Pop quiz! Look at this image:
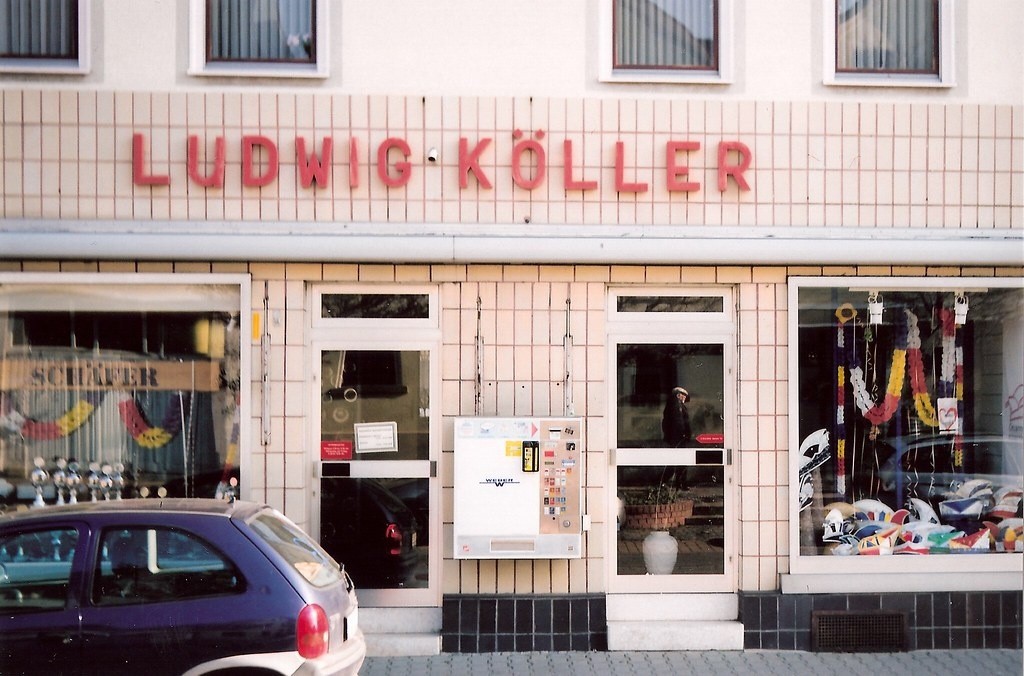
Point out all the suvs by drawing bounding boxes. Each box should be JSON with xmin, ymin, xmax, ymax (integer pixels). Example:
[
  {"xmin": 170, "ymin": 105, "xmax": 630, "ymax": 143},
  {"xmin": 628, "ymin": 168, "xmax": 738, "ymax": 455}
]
[{"xmin": 880, "ymin": 430, "xmax": 1023, "ymax": 505}]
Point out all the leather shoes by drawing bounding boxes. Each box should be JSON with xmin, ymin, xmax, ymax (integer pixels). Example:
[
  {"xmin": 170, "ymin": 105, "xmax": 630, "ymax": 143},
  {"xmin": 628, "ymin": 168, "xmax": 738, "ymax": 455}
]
[
  {"xmin": 661, "ymin": 483, "xmax": 674, "ymax": 491},
  {"xmin": 676, "ymin": 486, "xmax": 690, "ymax": 491}
]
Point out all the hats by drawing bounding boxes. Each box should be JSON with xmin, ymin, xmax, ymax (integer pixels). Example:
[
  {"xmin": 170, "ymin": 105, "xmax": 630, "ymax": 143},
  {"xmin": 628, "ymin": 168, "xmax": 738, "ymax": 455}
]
[{"xmin": 673, "ymin": 387, "xmax": 688, "ymax": 395}]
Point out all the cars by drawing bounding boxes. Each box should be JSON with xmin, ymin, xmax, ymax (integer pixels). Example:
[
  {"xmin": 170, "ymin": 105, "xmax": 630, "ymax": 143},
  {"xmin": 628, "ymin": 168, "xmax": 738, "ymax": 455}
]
[
  {"xmin": 0, "ymin": 499, "xmax": 367, "ymax": 676},
  {"xmin": 102, "ymin": 466, "xmax": 420, "ymax": 590}
]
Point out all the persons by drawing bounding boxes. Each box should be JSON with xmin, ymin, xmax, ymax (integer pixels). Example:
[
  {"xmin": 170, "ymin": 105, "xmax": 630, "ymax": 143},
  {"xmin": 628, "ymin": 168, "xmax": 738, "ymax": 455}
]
[{"xmin": 659, "ymin": 386, "xmax": 693, "ymax": 491}]
